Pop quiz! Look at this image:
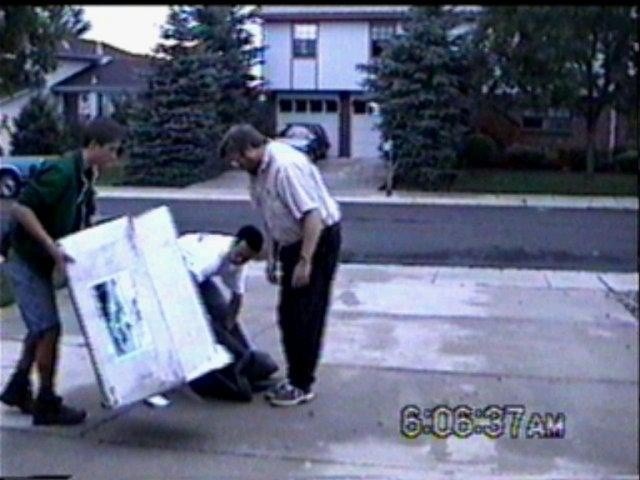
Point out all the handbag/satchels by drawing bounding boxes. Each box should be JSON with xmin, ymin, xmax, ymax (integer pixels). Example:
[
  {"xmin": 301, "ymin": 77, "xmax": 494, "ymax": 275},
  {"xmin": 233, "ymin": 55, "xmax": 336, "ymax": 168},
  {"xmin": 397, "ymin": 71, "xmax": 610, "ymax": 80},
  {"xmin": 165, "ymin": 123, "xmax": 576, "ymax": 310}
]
[{"xmin": 189, "ymin": 324, "xmax": 277, "ymax": 402}]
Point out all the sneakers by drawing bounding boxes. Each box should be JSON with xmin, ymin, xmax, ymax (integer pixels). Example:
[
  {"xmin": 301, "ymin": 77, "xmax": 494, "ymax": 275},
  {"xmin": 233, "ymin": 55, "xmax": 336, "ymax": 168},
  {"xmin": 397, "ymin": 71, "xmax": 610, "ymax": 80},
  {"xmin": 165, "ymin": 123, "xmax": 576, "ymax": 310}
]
[
  {"xmin": 143, "ymin": 395, "xmax": 172, "ymax": 408},
  {"xmin": 264, "ymin": 379, "xmax": 290, "ymax": 399},
  {"xmin": 32, "ymin": 389, "xmax": 86, "ymax": 425},
  {"xmin": 0, "ymin": 373, "xmax": 37, "ymax": 414},
  {"xmin": 271, "ymin": 384, "xmax": 314, "ymax": 407}
]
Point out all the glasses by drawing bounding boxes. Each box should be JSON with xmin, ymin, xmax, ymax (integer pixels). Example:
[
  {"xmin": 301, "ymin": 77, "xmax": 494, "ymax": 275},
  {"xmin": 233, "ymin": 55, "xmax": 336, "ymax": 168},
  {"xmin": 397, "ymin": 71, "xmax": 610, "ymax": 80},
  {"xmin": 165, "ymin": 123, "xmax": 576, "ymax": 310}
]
[{"xmin": 230, "ymin": 153, "xmax": 241, "ymax": 168}]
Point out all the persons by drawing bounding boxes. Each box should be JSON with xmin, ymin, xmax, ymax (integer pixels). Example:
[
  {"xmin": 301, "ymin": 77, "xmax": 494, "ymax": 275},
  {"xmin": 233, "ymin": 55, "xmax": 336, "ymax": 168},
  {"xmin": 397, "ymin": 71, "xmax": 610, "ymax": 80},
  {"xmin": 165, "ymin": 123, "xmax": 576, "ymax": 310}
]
[
  {"xmin": 1, "ymin": 117, "xmax": 123, "ymax": 426},
  {"xmin": 179, "ymin": 225, "xmax": 264, "ymax": 350},
  {"xmin": 218, "ymin": 123, "xmax": 343, "ymax": 408}
]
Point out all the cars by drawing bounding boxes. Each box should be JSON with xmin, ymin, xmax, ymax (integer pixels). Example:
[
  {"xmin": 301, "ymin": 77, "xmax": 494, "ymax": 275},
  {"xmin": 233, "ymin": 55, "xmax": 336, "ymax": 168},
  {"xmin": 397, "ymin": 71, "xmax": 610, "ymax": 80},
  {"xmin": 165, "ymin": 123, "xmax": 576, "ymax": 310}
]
[
  {"xmin": 0, "ymin": 155, "xmax": 62, "ymax": 199},
  {"xmin": 275, "ymin": 122, "xmax": 329, "ymax": 162}
]
